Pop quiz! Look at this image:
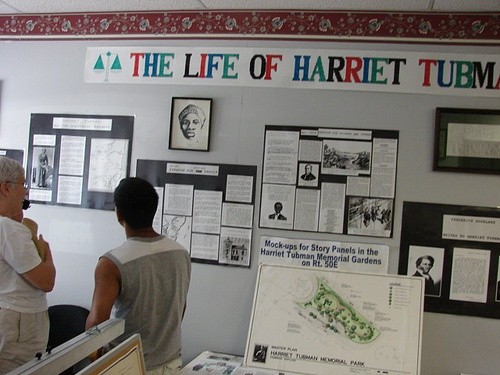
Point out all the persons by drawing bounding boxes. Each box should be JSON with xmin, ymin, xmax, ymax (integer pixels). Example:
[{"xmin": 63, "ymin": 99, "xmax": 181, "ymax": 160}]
[
  {"xmin": 86, "ymin": 177, "xmax": 191, "ymax": 375},
  {"xmin": 413, "ymin": 255, "xmax": 441, "ymax": 296},
  {"xmin": 269, "ymin": 202, "xmax": 287, "ymax": 220},
  {"xmin": 38, "ymin": 148, "xmax": 48, "ymax": 187},
  {"xmin": 349, "ymin": 205, "xmax": 391, "ymax": 227},
  {"xmin": 301, "ymin": 164, "xmax": 316, "ymax": 181},
  {"xmin": 0, "ymin": 156, "xmax": 56, "ymax": 375}
]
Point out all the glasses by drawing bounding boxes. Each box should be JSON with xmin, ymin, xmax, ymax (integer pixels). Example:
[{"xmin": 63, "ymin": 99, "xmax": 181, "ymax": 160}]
[{"xmin": 4, "ymin": 180, "xmax": 28, "ymax": 189}]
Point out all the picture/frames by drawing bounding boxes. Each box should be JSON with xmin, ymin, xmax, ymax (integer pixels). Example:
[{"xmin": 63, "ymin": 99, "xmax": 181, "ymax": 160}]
[{"xmin": 168, "ymin": 97, "xmax": 213, "ymax": 153}]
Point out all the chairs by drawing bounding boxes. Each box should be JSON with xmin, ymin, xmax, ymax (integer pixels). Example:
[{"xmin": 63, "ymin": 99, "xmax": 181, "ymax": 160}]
[{"xmin": 46, "ymin": 305, "xmax": 91, "ymax": 375}]
